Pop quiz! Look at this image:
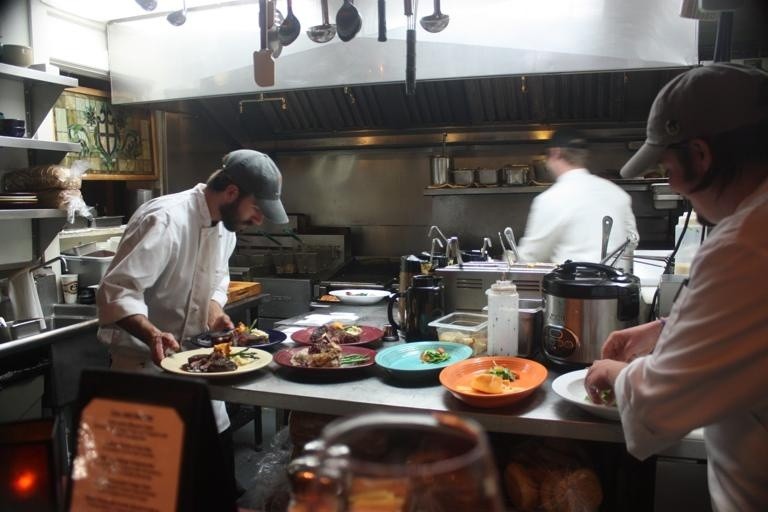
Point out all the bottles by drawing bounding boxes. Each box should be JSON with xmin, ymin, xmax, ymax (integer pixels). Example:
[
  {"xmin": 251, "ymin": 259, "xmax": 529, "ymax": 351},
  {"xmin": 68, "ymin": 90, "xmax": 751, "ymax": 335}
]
[
  {"xmin": 485, "ymin": 281, "xmax": 519, "ymax": 358},
  {"xmin": 673, "ymin": 211, "xmax": 707, "ymax": 275}
]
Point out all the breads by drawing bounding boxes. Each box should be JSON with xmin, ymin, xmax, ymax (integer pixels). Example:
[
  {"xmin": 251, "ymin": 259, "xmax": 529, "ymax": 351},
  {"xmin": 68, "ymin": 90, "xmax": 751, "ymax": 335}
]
[
  {"xmin": 4, "ymin": 165, "xmax": 69, "ymax": 190},
  {"xmin": 35, "ymin": 190, "xmax": 69, "ymax": 208}
]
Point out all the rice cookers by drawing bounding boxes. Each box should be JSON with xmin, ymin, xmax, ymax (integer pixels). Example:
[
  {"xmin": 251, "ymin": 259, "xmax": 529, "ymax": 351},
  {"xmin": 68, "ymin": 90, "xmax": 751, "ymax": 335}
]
[{"xmin": 541, "ymin": 261, "xmax": 641, "ymax": 371}]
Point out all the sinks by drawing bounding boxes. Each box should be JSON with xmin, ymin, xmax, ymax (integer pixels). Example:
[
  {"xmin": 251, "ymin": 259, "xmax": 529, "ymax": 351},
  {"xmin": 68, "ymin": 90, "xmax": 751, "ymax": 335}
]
[{"xmin": 0, "ymin": 317, "xmax": 89, "ymax": 344}]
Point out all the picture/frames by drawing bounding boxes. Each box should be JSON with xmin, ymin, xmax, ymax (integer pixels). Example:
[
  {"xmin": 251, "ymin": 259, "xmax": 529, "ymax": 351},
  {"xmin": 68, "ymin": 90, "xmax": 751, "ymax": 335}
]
[{"xmin": 53, "ymin": 85, "xmax": 159, "ymax": 183}]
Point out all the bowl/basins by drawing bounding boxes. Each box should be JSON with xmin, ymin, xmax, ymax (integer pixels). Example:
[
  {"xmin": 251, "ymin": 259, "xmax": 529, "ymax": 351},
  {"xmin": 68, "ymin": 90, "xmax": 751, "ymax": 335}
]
[
  {"xmin": 1, "ymin": 43, "xmax": 33, "ymax": 68},
  {"xmin": 2, "ymin": 118, "xmax": 25, "ymax": 136}
]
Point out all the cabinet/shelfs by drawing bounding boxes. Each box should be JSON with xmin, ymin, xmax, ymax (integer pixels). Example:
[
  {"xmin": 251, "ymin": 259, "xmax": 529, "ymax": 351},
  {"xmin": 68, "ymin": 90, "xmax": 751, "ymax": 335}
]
[{"xmin": 1, "ymin": 64, "xmax": 94, "ymax": 271}]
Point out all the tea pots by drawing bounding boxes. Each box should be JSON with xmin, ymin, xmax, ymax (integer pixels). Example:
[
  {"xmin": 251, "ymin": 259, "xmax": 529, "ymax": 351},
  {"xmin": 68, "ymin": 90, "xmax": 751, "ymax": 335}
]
[{"xmin": 387, "ymin": 274, "xmax": 444, "ymax": 342}]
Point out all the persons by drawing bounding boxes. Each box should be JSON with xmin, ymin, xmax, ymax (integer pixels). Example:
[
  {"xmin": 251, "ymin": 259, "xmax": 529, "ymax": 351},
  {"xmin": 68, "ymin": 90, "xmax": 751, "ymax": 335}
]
[
  {"xmin": 580, "ymin": 61, "xmax": 767, "ymax": 510},
  {"xmin": 506, "ymin": 125, "xmax": 642, "ymax": 273},
  {"xmin": 95, "ymin": 148, "xmax": 290, "ymax": 512}
]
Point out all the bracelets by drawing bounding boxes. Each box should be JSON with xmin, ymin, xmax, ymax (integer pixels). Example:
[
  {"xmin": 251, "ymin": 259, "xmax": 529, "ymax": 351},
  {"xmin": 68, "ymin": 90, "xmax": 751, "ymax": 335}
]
[{"xmin": 657, "ymin": 317, "xmax": 665, "ymax": 328}]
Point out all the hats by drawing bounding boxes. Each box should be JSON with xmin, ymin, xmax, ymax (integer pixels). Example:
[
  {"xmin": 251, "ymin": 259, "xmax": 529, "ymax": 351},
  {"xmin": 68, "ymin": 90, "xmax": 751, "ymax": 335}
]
[
  {"xmin": 544, "ymin": 128, "xmax": 586, "ymax": 149},
  {"xmin": 222, "ymin": 149, "xmax": 290, "ymax": 225},
  {"xmin": 619, "ymin": 62, "xmax": 767, "ymax": 179}
]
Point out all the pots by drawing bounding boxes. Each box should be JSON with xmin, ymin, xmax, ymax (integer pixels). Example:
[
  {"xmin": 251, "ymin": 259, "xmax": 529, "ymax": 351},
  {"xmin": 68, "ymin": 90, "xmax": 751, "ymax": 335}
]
[{"xmin": 421, "ymin": 150, "xmax": 554, "ymax": 186}]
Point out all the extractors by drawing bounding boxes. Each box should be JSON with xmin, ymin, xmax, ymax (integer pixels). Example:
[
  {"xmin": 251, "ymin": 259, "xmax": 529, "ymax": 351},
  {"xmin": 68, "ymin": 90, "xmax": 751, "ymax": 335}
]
[{"xmin": 105, "ymin": 0, "xmax": 700, "ymax": 152}]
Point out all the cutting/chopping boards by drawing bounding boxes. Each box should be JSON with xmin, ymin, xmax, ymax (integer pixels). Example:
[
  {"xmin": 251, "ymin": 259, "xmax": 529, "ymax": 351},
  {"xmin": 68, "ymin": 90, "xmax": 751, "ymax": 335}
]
[{"xmin": 221, "ymin": 280, "xmax": 262, "ymax": 306}]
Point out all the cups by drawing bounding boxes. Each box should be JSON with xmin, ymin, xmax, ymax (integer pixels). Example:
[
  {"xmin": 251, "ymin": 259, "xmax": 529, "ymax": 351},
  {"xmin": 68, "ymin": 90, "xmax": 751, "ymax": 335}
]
[
  {"xmin": 61, "ymin": 274, "xmax": 80, "ymax": 305},
  {"xmin": 318, "ymin": 412, "xmax": 500, "ymax": 512}
]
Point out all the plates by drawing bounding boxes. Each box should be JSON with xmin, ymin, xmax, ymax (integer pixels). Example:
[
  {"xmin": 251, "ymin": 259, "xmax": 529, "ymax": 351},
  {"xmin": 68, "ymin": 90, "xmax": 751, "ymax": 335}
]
[
  {"xmin": 551, "ymin": 368, "xmax": 621, "ymax": 422},
  {"xmin": 329, "ymin": 289, "xmax": 389, "ymax": 304},
  {"xmin": 273, "ymin": 346, "xmax": 377, "ymax": 373},
  {"xmin": 289, "ymin": 324, "xmax": 384, "ymax": 345},
  {"xmin": 159, "ymin": 346, "xmax": 273, "ymax": 379},
  {"xmin": 437, "ymin": 356, "xmax": 549, "ymax": 406},
  {"xmin": 191, "ymin": 327, "xmax": 286, "ymax": 351},
  {"xmin": 374, "ymin": 341, "xmax": 473, "ymax": 378}
]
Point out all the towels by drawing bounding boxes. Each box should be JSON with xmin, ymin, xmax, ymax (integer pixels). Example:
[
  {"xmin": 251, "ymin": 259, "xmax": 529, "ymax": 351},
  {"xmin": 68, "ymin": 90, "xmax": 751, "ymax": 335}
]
[{"xmin": 5, "ymin": 267, "xmax": 47, "ymax": 330}]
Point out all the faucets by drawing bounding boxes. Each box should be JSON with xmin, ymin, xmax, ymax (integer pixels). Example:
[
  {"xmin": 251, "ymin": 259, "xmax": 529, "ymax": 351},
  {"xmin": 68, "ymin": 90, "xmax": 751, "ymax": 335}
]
[{"xmin": 1, "ymin": 255, "xmax": 68, "ymax": 298}]
